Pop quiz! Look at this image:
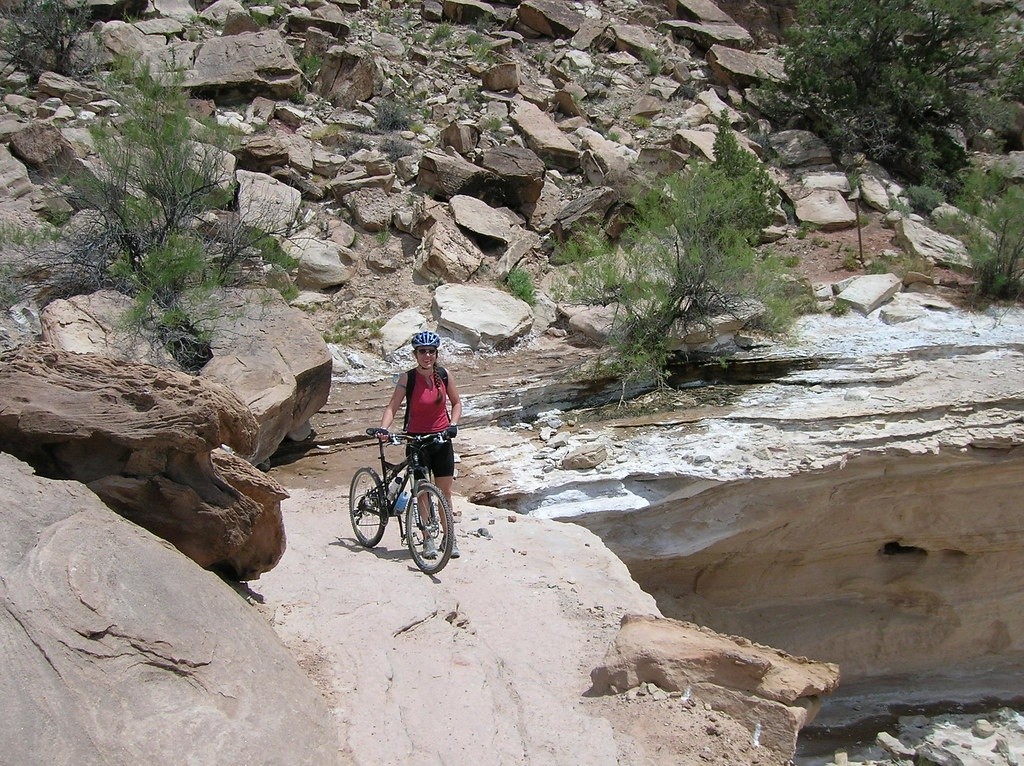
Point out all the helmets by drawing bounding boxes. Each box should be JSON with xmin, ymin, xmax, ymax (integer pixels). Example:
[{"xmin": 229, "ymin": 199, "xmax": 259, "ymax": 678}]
[{"xmin": 412, "ymin": 331, "xmax": 440, "ymax": 350}]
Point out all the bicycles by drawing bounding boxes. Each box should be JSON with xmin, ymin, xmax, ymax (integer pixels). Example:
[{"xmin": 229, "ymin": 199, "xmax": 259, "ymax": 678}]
[{"xmin": 347, "ymin": 428, "xmax": 455, "ymax": 576}]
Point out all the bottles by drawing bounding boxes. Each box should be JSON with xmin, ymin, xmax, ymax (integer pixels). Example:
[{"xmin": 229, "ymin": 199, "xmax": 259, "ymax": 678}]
[
  {"xmin": 394, "ymin": 491, "xmax": 409, "ymax": 514},
  {"xmin": 387, "ymin": 477, "xmax": 402, "ymax": 500}
]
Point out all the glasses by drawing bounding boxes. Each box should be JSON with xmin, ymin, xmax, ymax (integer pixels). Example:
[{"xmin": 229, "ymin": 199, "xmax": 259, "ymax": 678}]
[{"xmin": 414, "ymin": 349, "xmax": 437, "ymax": 354}]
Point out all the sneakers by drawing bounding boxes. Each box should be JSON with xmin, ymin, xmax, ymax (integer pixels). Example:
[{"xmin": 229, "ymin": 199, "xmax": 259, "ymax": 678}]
[
  {"xmin": 422, "ymin": 537, "xmax": 438, "ymax": 560},
  {"xmin": 439, "ymin": 537, "xmax": 460, "ymax": 559}
]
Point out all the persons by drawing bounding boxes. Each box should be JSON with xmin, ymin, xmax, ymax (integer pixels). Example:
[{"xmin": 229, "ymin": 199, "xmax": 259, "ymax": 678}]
[{"xmin": 375, "ymin": 331, "xmax": 462, "ymax": 557}]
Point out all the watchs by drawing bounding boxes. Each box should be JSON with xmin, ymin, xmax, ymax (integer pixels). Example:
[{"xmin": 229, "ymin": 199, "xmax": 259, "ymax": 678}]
[{"xmin": 449, "ymin": 422, "xmax": 457, "ymax": 426}]
